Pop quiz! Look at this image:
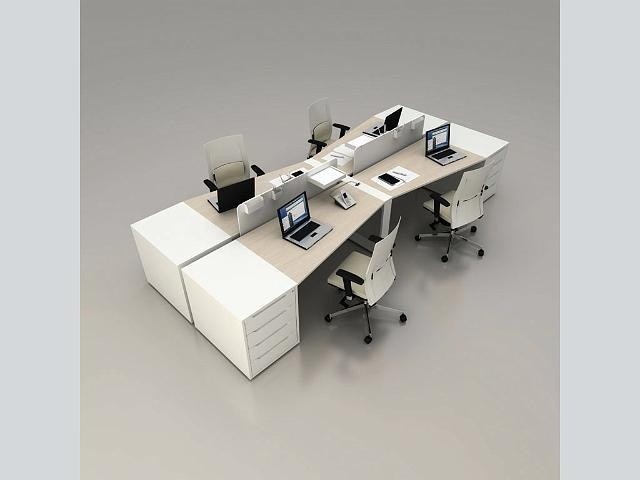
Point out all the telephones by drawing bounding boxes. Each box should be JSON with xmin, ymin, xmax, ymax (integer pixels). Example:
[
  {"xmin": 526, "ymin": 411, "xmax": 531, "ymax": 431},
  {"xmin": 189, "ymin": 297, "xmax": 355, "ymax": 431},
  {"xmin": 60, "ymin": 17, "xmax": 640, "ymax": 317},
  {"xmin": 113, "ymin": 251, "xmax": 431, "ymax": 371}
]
[{"xmin": 330, "ymin": 188, "xmax": 356, "ymax": 210}]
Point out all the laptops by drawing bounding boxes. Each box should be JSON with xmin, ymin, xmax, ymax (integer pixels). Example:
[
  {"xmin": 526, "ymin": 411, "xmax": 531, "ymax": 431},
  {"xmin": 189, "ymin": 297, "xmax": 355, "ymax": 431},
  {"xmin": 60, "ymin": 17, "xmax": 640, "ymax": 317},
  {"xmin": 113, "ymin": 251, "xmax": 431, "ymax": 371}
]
[
  {"xmin": 276, "ymin": 190, "xmax": 334, "ymax": 250},
  {"xmin": 425, "ymin": 122, "xmax": 468, "ymax": 166},
  {"xmin": 207, "ymin": 176, "xmax": 256, "ymax": 214},
  {"xmin": 362, "ymin": 106, "xmax": 403, "ymax": 137}
]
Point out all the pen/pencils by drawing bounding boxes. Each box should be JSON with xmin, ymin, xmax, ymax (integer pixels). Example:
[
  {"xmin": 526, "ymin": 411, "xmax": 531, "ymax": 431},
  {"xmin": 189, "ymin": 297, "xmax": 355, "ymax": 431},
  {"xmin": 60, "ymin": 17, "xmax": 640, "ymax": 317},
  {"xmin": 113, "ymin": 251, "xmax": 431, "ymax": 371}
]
[
  {"xmin": 392, "ymin": 171, "xmax": 406, "ymax": 176},
  {"xmin": 331, "ymin": 155, "xmax": 344, "ymax": 158}
]
